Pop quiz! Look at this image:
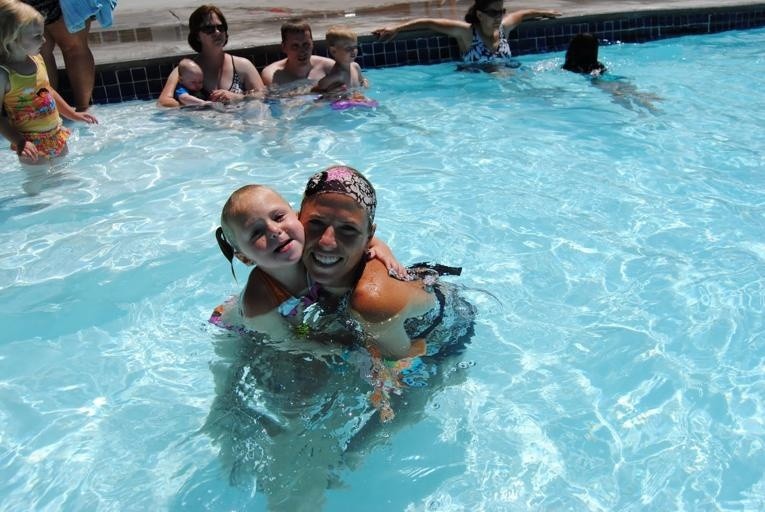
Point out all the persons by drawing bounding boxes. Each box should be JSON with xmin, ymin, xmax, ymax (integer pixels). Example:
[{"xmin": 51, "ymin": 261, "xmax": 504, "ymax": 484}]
[
  {"xmin": 159, "ymin": 5, "xmax": 266, "ymax": 107},
  {"xmin": 376, "ymin": 2, "xmax": 563, "ymax": 74},
  {"xmin": 559, "ymin": 36, "xmax": 668, "ymax": 123},
  {"xmin": 172, "ymin": 58, "xmax": 216, "ymax": 109},
  {"xmin": 38, "ymin": 1, "xmax": 117, "ymax": 110},
  {"xmin": 295, "ymin": 163, "xmax": 476, "ymax": 357},
  {"xmin": 315, "ymin": 27, "xmax": 370, "ymax": 93},
  {"xmin": 260, "ymin": 18, "xmax": 349, "ymax": 97},
  {"xmin": 1, "ymin": 1, "xmax": 99, "ymax": 166},
  {"xmin": 208, "ymin": 187, "xmax": 398, "ymax": 424}
]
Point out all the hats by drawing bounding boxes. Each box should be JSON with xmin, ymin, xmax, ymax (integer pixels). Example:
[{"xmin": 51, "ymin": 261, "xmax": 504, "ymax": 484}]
[{"xmin": 303, "ymin": 165, "xmax": 378, "ymax": 228}]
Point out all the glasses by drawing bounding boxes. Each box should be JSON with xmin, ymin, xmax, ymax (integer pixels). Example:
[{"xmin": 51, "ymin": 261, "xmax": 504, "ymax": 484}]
[
  {"xmin": 199, "ymin": 23, "xmax": 229, "ymax": 34},
  {"xmin": 478, "ymin": 6, "xmax": 506, "ymax": 18}
]
